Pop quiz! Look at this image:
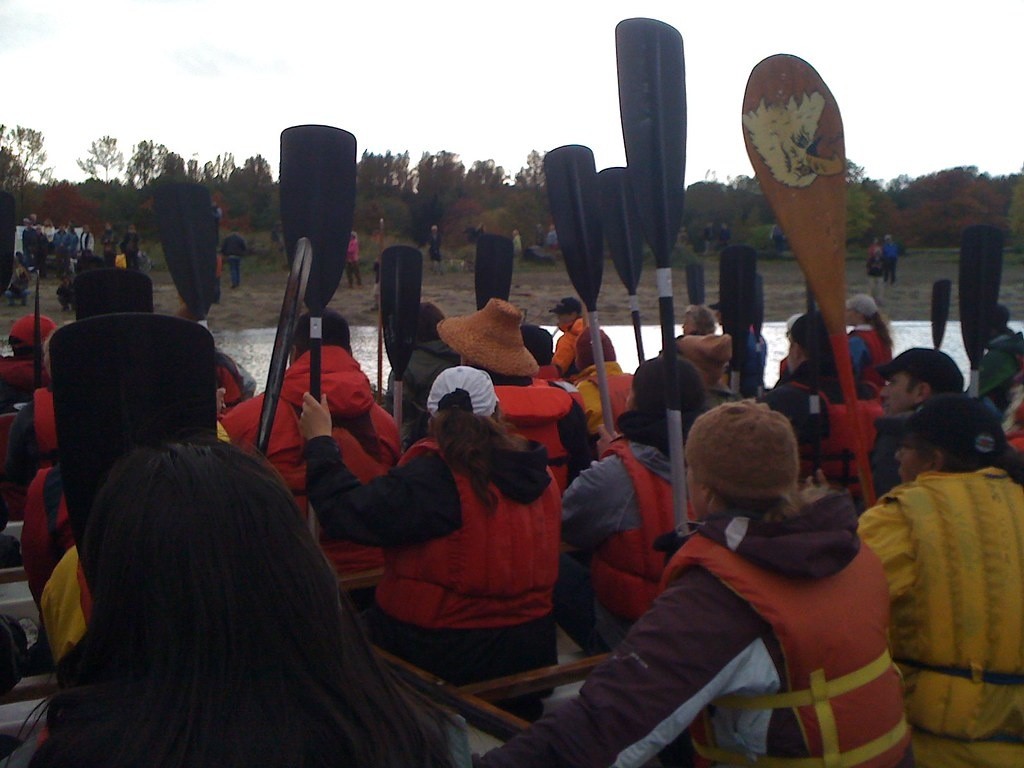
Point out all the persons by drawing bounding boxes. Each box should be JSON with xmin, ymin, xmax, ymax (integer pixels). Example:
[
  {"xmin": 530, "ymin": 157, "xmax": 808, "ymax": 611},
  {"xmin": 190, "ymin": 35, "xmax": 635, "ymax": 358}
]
[
  {"xmin": 427, "ymin": 225, "xmax": 444, "ymax": 272},
  {"xmin": 5, "ymin": 210, "xmax": 140, "ymax": 312},
  {"xmin": 346, "ymin": 231, "xmax": 363, "ymax": 289},
  {"xmin": 535, "ymin": 223, "xmax": 558, "ymax": 250},
  {"xmin": 510, "ymin": 227, "xmax": 524, "ymax": 267},
  {"xmin": 461, "ymin": 220, "xmax": 488, "ymax": 244},
  {"xmin": 865, "ymin": 234, "xmax": 899, "ymax": 285},
  {"xmin": 0, "ymin": 293, "xmax": 1024, "ymax": 768},
  {"xmin": 214, "ymin": 226, "xmax": 246, "ymax": 305},
  {"xmin": 771, "ymin": 218, "xmax": 785, "ymax": 251},
  {"xmin": 702, "ymin": 221, "xmax": 731, "ymax": 256}
]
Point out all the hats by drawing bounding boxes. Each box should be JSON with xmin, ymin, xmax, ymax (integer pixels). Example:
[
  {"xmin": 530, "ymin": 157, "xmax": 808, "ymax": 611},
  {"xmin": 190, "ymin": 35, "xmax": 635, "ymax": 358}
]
[
  {"xmin": 549, "ymin": 297, "xmax": 582, "ymax": 313},
  {"xmin": 792, "ymin": 310, "xmax": 827, "ymax": 345},
  {"xmin": 576, "ymin": 326, "xmax": 616, "ymax": 370},
  {"xmin": 876, "ymin": 348, "xmax": 964, "ymax": 393},
  {"xmin": 993, "ymin": 303, "xmax": 1008, "ymax": 323},
  {"xmin": 416, "ymin": 303, "xmax": 445, "ymax": 340},
  {"xmin": 521, "ymin": 325, "xmax": 553, "ymax": 364},
  {"xmin": 632, "ymin": 354, "xmax": 702, "ymax": 414},
  {"xmin": 885, "ymin": 235, "xmax": 891, "ymax": 240},
  {"xmin": 677, "ymin": 333, "xmax": 731, "ymax": 386},
  {"xmin": 9, "ymin": 315, "xmax": 58, "ymax": 348},
  {"xmin": 437, "ymin": 298, "xmax": 540, "ymax": 375},
  {"xmin": 294, "ymin": 307, "xmax": 351, "ymax": 343},
  {"xmin": 845, "ymin": 294, "xmax": 877, "ymax": 318},
  {"xmin": 874, "ymin": 394, "xmax": 1006, "ymax": 454},
  {"xmin": 428, "ymin": 366, "xmax": 499, "ymax": 416},
  {"xmin": 686, "ymin": 397, "xmax": 800, "ymax": 499}
]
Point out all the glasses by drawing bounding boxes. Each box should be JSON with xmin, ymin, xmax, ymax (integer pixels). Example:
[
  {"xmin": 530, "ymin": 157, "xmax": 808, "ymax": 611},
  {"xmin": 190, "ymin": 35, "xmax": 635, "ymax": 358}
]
[{"xmin": 786, "ymin": 330, "xmax": 791, "ymax": 337}]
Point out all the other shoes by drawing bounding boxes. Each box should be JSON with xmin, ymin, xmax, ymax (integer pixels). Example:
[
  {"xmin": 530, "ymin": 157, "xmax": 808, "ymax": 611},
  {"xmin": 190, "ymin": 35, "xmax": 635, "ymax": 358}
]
[{"xmin": 14, "ymin": 642, "xmax": 55, "ymax": 677}]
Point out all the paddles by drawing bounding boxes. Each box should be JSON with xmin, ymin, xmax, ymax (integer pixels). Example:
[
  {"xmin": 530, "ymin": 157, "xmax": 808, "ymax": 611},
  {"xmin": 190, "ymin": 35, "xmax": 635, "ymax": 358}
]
[
  {"xmin": 931, "ymin": 280, "xmax": 951, "ymax": 351},
  {"xmin": 153, "ymin": 181, "xmax": 218, "ymax": 328},
  {"xmin": 255, "ymin": 237, "xmax": 314, "ymax": 458},
  {"xmin": 474, "ymin": 234, "xmax": 514, "ymax": 310},
  {"xmin": 0, "ymin": 191, "xmax": 16, "ymax": 295},
  {"xmin": 741, "ymin": 53, "xmax": 877, "ymax": 507},
  {"xmin": 279, "ymin": 124, "xmax": 357, "ymax": 544},
  {"xmin": 751, "ymin": 274, "xmax": 764, "ymax": 398},
  {"xmin": 73, "ymin": 267, "xmax": 153, "ymax": 321},
  {"xmin": 543, "ymin": 145, "xmax": 615, "ymax": 439},
  {"xmin": 380, "ymin": 245, "xmax": 423, "ymax": 434},
  {"xmin": 806, "ymin": 280, "xmax": 821, "ymax": 488},
  {"xmin": 49, "ymin": 313, "xmax": 218, "ymax": 604},
  {"xmin": 719, "ymin": 245, "xmax": 757, "ymax": 394},
  {"xmin": 958, "ymin": 223, "xmax": 1004, "ymax": 398},
  {"xmin": 597, "ymin": 167, "xmax": 646, "ymax": 366},
  {"xmin": 685, "ymin": 262, "xmax": 705, "ymax": 305},
  {"xmin": 34, "ymin": 276, "xmax": 41, "ymax": 388},
  {"xmin": 614, "ymin": 16, "xmax": 687, "ymax": 538}
]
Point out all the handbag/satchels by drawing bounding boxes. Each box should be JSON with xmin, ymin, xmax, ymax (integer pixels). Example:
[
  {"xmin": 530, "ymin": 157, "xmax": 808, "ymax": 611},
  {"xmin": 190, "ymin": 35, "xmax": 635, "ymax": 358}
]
[{"xmin": 114, "ymin": 253, "xmax": 127, "ymax": 269}]
